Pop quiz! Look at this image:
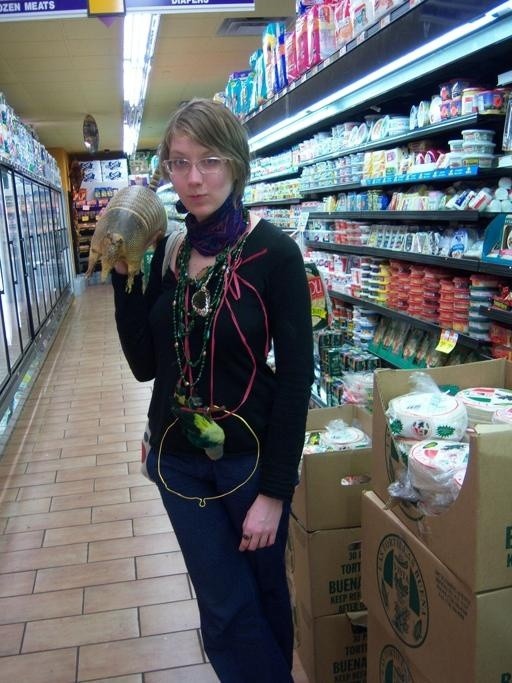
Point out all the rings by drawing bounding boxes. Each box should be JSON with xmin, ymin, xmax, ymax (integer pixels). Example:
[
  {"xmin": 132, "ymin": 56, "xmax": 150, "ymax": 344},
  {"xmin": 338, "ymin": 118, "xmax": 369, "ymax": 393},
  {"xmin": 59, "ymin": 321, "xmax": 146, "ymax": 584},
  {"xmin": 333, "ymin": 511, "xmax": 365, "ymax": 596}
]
[{"xmin": 241, "ymin": 533, "xmax": 250, "ymax": 541}]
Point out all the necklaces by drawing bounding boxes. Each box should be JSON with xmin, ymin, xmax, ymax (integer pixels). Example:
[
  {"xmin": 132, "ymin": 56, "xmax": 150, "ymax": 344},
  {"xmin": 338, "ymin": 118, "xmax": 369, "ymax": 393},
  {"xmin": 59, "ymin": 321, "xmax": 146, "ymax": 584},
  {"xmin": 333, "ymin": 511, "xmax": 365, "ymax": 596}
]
[{"xmin": 172, "ymin": 206, "xmax": 252, "ymax": 393}]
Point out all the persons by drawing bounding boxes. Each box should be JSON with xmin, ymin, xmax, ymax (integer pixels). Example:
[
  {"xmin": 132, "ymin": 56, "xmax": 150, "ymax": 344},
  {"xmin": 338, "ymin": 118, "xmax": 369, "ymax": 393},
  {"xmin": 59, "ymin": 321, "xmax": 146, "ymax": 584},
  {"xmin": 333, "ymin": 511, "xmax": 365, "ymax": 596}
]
[{"xmin": 109, "ymin": 94, "xmax": 320, "ymax": 682}]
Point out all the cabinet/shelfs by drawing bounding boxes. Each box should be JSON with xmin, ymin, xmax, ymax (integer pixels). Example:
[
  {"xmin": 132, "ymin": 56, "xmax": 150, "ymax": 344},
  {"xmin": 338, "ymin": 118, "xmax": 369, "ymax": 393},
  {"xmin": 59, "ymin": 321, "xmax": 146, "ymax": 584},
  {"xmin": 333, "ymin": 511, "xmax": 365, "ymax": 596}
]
[
  {"xmin": 0, "ymin": 157, "xmax": 80, "ymax": 459},
  {"xmin": 72, "ymin": 185, "xmax": 119, "ymax": 283}
]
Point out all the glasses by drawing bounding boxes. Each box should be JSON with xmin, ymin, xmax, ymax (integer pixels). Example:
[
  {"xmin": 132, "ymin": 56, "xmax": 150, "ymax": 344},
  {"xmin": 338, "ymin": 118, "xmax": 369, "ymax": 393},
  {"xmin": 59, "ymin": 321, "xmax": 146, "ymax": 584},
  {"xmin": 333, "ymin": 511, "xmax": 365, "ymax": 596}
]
[{"xmin": 162, "ymin": 156, "xmax": 228, "ymax": 175}]
